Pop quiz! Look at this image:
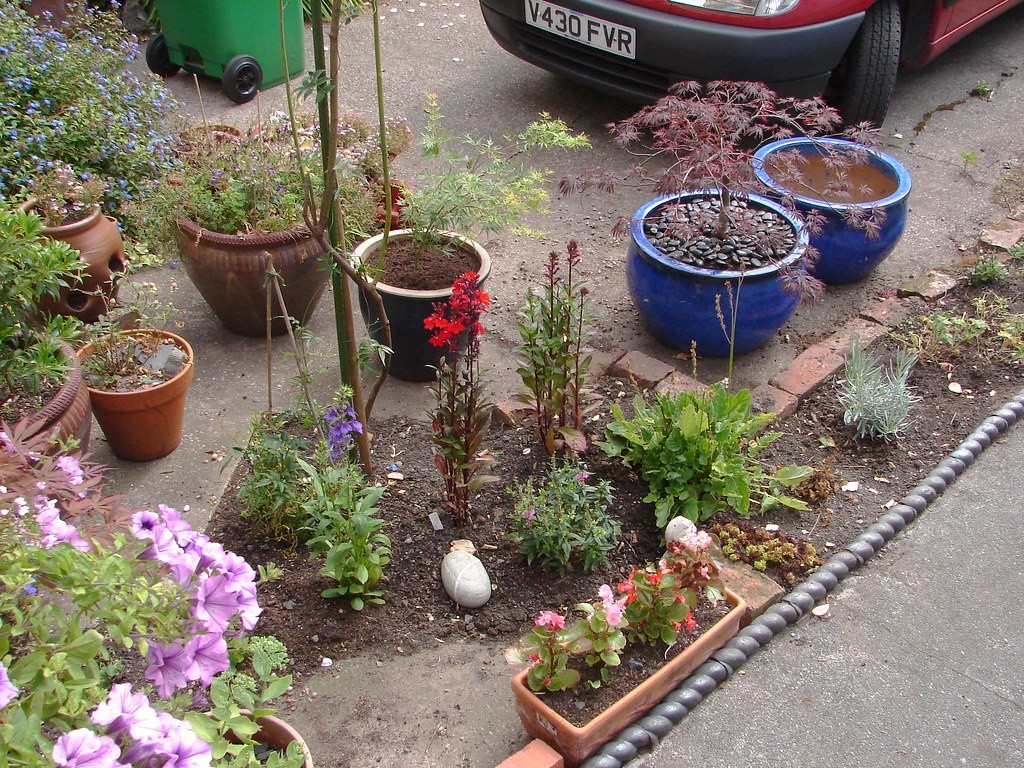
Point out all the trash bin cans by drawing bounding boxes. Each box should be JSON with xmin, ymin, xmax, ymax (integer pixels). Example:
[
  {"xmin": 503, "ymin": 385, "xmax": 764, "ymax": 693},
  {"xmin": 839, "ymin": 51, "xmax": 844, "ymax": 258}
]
[{"xmin": 145, "ymin": 0, "xmax": 305, "ymax": 102}]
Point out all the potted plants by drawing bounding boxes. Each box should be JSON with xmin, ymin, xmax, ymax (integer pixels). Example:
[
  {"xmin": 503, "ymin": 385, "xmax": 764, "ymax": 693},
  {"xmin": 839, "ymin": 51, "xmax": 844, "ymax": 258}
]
[
  {"xmin": 0, "ymin": 208, "xmax": 95, "ymax": 475},
  {"xmin": 358, "ymin": 90, "xmax": 594, "ymax": 380},
  {"xmin": 559, "ymin": 79, "xmax": 884, "ymax": 360}
]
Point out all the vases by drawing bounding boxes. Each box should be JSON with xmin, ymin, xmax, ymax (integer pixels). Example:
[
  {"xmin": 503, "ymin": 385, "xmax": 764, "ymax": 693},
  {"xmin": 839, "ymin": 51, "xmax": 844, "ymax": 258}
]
[
  {"xmin": 510, "ymin": 571, "xmax": 748, "ymax": 764},
  {"xmin": 192, "ymin": 708, "xmax": 315, "ymax": 768},
  {"xmin": 18, "ymin": 191, "xmax": 127, "ymax": 326},
  {"xmin": 170, "ymin": 198, "xmax": 334, "ymax": 338},
  {"xmin": 752, "ymin": 137, "xmax": 911, "ymax": 287},
  {"xmin": 77, "ymin": 328, "xmax": 194, "ymax": 462}
]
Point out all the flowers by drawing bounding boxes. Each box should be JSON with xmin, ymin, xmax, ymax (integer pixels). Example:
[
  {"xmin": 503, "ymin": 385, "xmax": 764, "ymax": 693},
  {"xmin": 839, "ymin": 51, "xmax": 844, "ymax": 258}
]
[
  {"xmin": 522, "ymin": 522, "xmax": 718, "ymax": 691},
  {"xmin": 20, "ymin": 162, "xmax": 112, "ymax": 226}
]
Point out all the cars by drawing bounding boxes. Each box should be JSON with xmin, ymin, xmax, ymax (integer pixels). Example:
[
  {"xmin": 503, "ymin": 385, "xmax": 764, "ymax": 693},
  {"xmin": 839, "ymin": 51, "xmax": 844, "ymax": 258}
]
[{"xmin": 479, "ymin": 0, "xmax": 1023, "ymax": 144}]
